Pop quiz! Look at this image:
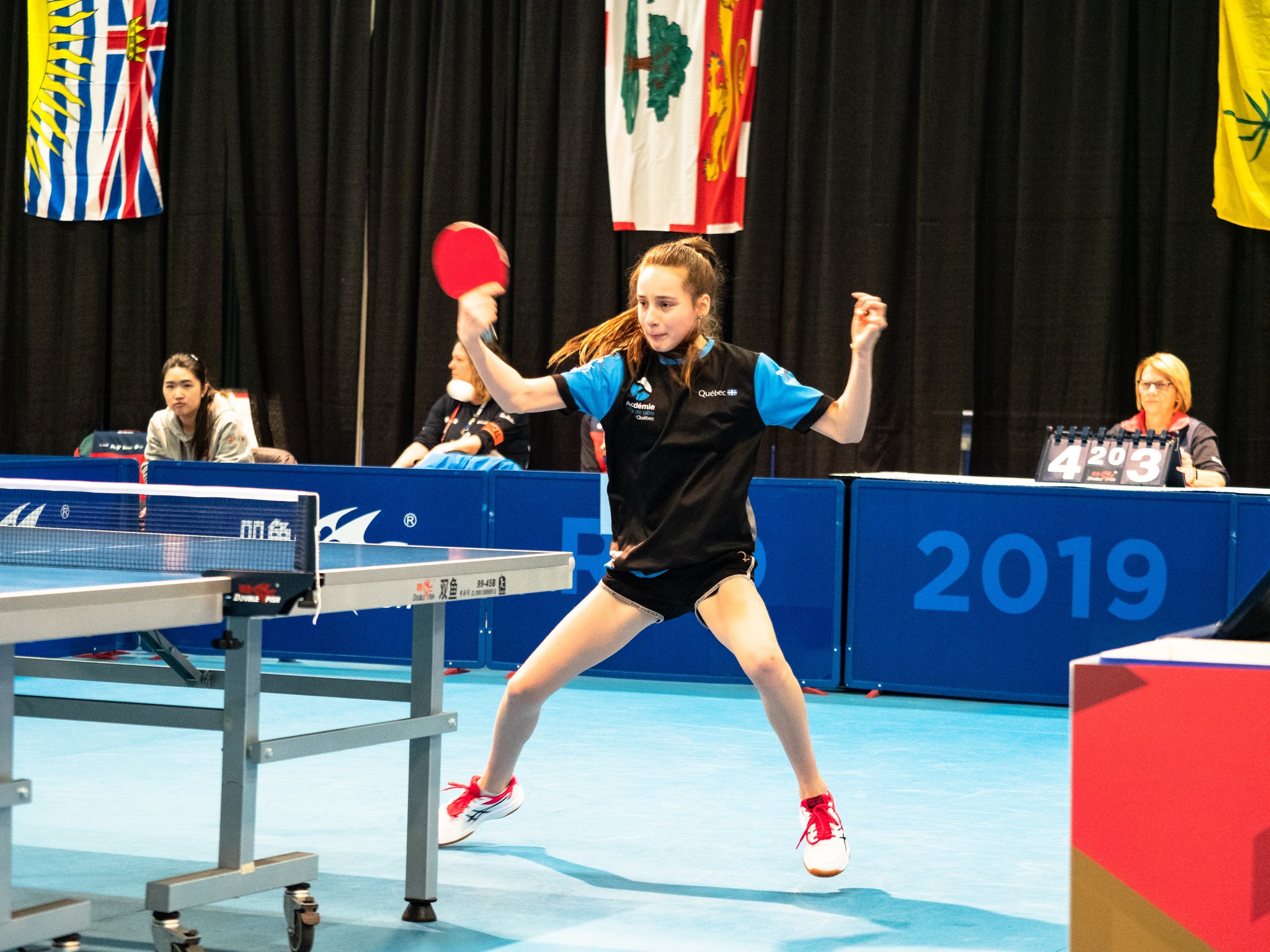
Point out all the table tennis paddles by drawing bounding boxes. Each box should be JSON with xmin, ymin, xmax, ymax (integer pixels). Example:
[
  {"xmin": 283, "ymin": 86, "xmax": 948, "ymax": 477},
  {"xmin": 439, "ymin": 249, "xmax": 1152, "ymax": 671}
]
[{"xmin": 430, "ymin": 219, "xmax": 512, "ymax": 345}]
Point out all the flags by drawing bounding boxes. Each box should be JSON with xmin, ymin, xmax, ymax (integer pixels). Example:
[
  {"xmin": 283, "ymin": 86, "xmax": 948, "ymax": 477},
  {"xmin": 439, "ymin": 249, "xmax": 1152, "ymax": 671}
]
[
  {"xmin": 604, "ymin": 0, "xmax": 763, "ymax": 235},
  {"xmin": 1210, "ymin": 0, "xmax": 1270, "ymax": 230},
  {"xmin": 23, "ymin": 0, "xmax": 167, "ymax": 221}
]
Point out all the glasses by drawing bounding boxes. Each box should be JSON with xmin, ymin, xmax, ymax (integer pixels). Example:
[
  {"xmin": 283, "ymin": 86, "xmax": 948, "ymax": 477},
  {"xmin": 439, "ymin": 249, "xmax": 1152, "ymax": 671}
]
[{"xmin": 1137, "ymin": 380, "xmax": 1174, "ymax": 391}]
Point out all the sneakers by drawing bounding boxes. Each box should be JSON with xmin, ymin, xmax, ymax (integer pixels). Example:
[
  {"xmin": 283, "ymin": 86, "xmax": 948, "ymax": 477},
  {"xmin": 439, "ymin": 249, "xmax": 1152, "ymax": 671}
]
[
  {"xmin": 438, "ymin": 774, "xmax": 524, "ymax": 846},
  {"xmin": 800, "ymin": 790, "xmax": 851, "ymax": 878}
]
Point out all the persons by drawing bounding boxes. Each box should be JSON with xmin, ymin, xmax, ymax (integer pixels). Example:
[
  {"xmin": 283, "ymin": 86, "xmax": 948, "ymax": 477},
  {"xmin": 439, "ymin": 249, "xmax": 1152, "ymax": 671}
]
[
  {"xmin": 437, "ymin": 235, "xmax": 887, "ymax": 877},
  {"xmin": 139, "ymin": 352, "xmax": 254, "ymax": 532},
  {"xmin": 390, "ymin": 338, "xmax": 528, "ymax": 470},
  {"xmin": 579, "ymin": 415, "xmax": 608, "ymax": 473},
  {"xmin": 1103, "ymin": 352, "xmax": 1230, "ymax": 488}
]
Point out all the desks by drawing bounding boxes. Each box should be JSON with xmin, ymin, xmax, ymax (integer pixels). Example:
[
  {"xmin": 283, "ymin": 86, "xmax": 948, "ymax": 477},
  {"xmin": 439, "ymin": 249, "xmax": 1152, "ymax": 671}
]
[{"xmin": 0, "ymin": 446, "xmax": 1269, "ymax": 706}]
